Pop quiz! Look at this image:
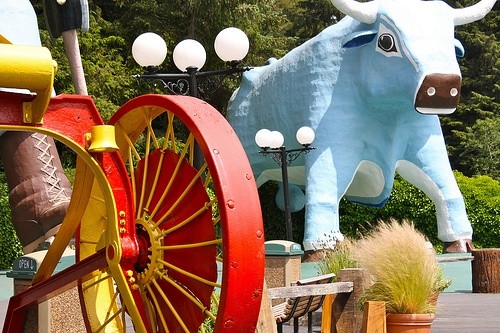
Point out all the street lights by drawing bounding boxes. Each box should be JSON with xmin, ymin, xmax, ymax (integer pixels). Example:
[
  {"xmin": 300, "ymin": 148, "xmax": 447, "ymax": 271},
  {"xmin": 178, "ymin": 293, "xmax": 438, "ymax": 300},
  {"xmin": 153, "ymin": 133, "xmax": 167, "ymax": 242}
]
[
  {"xmin": 255, "ymin": 125, "xmax": 317, "ymax": 243},
  {"xmin": 130, "ymin": 27, "xmax": 255, "ymax": 185}
]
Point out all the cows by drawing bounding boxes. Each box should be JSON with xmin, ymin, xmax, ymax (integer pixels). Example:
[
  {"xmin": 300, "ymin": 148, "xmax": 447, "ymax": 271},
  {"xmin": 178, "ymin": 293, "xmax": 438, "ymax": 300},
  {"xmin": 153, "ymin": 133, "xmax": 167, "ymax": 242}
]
[{"xmin": 217, "ymin": 0, "xmax": 497, "ymax": 264}]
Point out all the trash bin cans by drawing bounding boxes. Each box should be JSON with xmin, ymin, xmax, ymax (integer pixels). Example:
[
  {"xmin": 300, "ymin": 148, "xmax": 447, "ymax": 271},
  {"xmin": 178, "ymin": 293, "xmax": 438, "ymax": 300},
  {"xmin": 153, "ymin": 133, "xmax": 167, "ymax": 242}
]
[
  {"xmin": 263, "ymin": 239, "xmax": 305, "ymax": 307},
  {"xmin": 4, "ymin": 248, "xmax": 77, "ymax": 332}
]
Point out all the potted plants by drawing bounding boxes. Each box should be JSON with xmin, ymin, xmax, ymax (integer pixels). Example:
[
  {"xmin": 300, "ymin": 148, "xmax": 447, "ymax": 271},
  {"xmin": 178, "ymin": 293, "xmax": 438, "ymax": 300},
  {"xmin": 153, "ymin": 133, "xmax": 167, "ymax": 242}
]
[{"xmin": 308, "ymin": 217, "xmax": 451, "ymax": 333}]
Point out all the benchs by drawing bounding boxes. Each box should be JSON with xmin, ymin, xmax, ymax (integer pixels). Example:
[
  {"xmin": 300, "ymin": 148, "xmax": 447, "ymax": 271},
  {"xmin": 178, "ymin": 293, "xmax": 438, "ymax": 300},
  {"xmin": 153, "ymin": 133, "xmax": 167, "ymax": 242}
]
[{"xmin": 272, "ymin": 273, "xmax": 336, "ymax": 333}]
